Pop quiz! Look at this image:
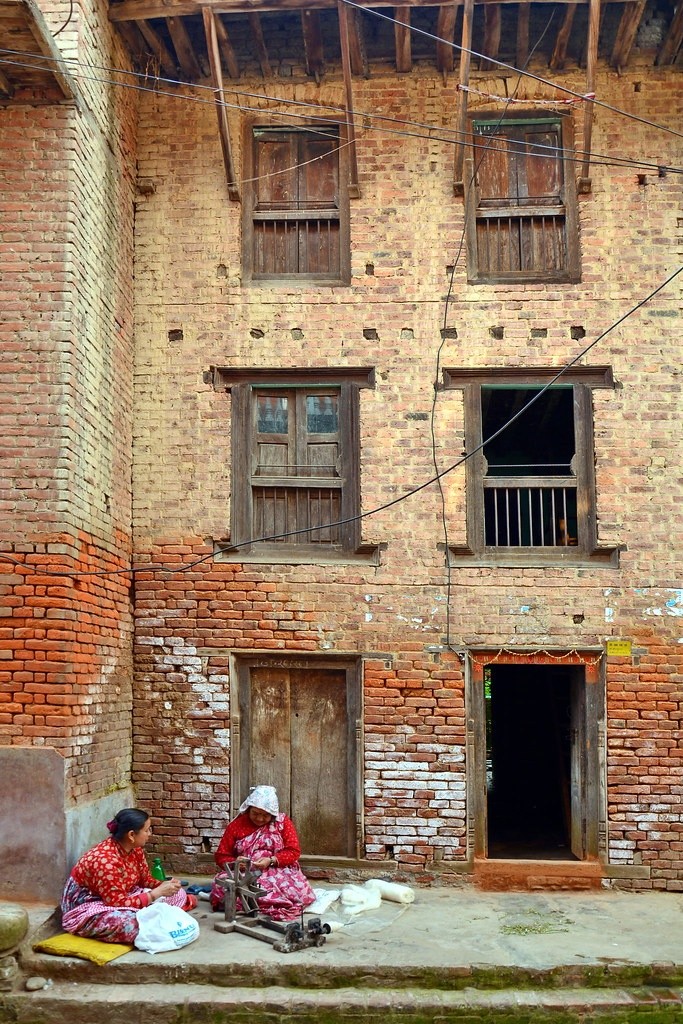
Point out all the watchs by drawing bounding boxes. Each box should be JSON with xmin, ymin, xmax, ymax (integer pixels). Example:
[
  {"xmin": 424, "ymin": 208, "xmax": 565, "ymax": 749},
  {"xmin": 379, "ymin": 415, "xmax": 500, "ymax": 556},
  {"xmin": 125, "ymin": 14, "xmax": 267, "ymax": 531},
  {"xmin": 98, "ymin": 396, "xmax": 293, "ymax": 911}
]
[{"xmin": 269, "ymin": 856, "xmax": 274, "ymax": 866}]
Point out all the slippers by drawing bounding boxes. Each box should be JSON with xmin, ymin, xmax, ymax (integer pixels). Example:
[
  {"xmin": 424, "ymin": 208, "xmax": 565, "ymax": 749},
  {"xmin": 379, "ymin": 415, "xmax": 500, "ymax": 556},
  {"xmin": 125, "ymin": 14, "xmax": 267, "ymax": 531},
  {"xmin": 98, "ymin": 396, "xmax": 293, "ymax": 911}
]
[
  {"xmin": 166, "ymin": 877, "xmax": 189, "ymax": 886},
  {"xmin": 186, "ymin": 885, "xmax": 212, "ymax": 894}
]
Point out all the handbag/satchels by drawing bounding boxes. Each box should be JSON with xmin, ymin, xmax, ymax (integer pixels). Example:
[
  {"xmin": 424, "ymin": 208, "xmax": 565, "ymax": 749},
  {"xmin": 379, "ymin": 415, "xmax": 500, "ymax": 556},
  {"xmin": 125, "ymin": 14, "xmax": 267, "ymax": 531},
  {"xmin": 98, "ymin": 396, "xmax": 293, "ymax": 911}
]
[{"xmin": 134, "ymin": 902, "xmax": 200, "ymax": 956}]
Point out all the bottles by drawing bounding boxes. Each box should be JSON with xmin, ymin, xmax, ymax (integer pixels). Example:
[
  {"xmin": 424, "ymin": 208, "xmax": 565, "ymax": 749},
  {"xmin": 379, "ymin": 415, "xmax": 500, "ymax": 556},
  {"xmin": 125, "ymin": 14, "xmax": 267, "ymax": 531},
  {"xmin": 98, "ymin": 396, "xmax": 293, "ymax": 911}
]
[{"xmin": 152, "ymin": 858, "xmax": 165, "ymax": 881}]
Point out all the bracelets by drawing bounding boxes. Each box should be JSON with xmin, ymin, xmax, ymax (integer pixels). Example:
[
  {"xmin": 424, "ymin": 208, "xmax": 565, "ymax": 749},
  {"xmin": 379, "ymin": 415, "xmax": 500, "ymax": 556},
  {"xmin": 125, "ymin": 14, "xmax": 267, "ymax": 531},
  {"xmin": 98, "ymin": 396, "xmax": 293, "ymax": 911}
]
[{"xmin": 148, "ymin": 890, "xmax": 156, "ymax": 902}]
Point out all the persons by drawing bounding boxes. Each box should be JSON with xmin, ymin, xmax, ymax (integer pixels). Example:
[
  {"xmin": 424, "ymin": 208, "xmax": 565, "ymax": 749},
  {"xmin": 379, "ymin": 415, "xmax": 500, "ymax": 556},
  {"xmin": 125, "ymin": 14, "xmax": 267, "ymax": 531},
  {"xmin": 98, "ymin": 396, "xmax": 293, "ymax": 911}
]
[
  {"xmin": 62, "ymin": 807, "xmax": 188, "ymax": 946},
  {"xmin": 210, "ymin": 786, "xmax": 316, "ymax": 921}
]
